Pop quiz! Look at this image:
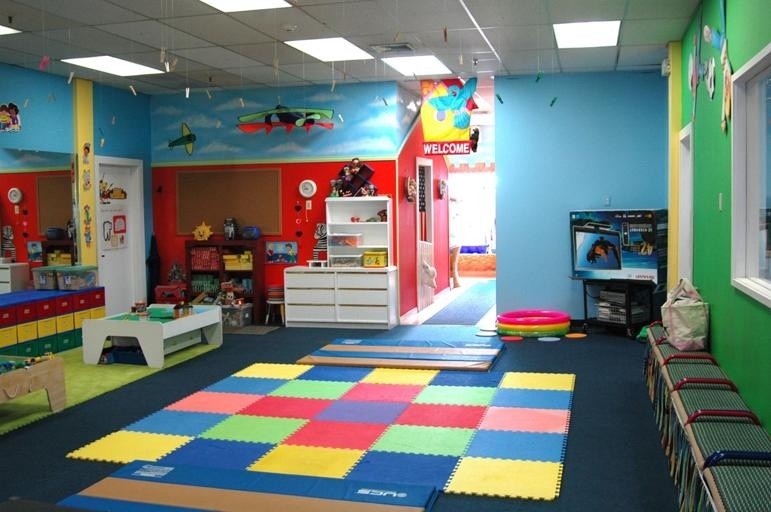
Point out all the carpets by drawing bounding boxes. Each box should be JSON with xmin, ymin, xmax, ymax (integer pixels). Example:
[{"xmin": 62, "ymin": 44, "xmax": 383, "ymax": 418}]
[{"xmin": 0, "ymin": 340, "xmax": 225, "ymax": 436}]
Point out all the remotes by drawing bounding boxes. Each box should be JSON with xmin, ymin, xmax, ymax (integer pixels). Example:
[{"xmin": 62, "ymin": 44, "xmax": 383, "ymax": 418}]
[{"xmin": 622, "ymin": 222, "xmax": 629, "ymax": 246}]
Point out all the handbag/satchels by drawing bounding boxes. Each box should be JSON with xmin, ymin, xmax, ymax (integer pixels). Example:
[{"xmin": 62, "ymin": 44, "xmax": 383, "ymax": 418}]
[{"xmin": 661, "ymin": 278, "xmax": 710, "ymax": 351}]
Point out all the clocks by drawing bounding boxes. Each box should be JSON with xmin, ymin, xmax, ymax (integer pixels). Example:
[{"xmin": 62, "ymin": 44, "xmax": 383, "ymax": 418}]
[
  {"xmin": 8, "ymin": 188, "xmax": 23, "ymax": 204},
  {"xmin": 299, "ymin": 178, "xmax": 318, "ymax": 198}
]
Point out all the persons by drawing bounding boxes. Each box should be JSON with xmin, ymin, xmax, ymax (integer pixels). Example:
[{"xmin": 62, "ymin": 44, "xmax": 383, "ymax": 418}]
[{"xmin": 329, "ymin": 157, "xmax": 377, "ymax": 197}]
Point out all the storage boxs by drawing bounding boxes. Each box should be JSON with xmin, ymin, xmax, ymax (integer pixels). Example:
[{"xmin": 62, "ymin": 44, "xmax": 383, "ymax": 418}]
[
  {"xmin": 219, "ymin": 303, "xmax": 254, "ymax": 326},
  {"xmin": 31, "ymin": 265, "xmax": 58, "ymax": 291},
  {"xmin": 569, "ymin": 210, "xmax": 669, "ymax": 284},
  {"xmin": 55, "ymin": 265, "xmax": 99, "ymax": 292}
]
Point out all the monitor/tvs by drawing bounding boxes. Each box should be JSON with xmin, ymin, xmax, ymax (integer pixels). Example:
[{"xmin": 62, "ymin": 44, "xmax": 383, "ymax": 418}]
[{"xmin": 572, "ymin": 223, "xmax": 621, "ymax": 270}]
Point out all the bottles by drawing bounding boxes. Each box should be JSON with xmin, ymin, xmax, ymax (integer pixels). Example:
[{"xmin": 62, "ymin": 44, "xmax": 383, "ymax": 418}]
[
  {"xmin": 382, "ymin": 215, "xmax": 387, "ymax": 221},
  {"xmin": 224, "ymin": 218, "xmax": 237, "ymax": 240},
  {"xmin": 239, "ymin": 226, "xmax": 261, "ymax": 241}
]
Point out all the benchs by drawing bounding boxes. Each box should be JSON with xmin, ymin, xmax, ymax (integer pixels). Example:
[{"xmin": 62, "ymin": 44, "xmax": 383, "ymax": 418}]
[{"xmin": 644, "ymin": 321, "xmax": 771, "ymax": 511}]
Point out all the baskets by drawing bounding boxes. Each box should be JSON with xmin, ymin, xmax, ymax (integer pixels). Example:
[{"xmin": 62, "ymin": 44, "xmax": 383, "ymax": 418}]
[{"xmin": 594, "ymin": 302, "xmax": 651, "ymax": 325}]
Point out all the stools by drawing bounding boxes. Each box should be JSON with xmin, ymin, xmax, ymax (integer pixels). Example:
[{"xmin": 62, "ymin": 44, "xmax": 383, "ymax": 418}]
[{"xmin": 266, "ymin": 286, "xmax": 286, "ymax": 328}]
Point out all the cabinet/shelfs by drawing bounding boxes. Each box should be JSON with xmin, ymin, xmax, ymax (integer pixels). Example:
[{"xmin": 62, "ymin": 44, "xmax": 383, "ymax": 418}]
[
  {"xmin": 185, "ymin": 239, "xmax": 266, "ymax": 323},
  {"xmin": 0, "ymin": 288, "xmax": 106, "ymax": 374},
  {"xmin": 282, "ymin": 196, "xmax": 397, "ymax": 329},
  {"xmin": 0, "ymin": 262, "xmax": 29, "ymax": 293}
]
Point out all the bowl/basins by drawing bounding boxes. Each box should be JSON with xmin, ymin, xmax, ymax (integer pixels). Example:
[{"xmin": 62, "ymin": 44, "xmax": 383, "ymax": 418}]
[{"xmin": 637, "ymin": 325, "xmax": 650, "ymax": 341}]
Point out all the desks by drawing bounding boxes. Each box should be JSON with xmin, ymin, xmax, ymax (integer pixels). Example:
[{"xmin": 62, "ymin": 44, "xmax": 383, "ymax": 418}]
[{"xmin": 0, "ymin": 303, "xmax": 224, "ymax": 411}]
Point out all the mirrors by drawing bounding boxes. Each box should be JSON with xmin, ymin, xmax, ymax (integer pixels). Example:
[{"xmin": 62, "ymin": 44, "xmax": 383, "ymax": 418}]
[{"xmin": 1, "ymin": 148, "xmax": 78, "ymax": 264}]
